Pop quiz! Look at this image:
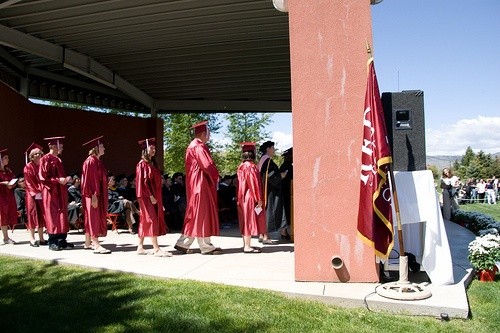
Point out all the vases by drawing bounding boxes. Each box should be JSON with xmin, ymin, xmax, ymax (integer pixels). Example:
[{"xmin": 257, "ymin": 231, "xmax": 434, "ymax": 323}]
[{"xmin": 479, "ymin": 266, "xmax": 496, "ymax": 281}]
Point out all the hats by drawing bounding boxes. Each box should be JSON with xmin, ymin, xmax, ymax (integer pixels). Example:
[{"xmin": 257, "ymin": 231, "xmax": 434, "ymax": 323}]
[
  {"xmin": 190, "ymin": 120, "xmax": 209, "ymax": 140},
  {"xmin": 0, "ymin": 147, "xmax": 9, "ymax": 157},
  {"xmin": 82, "ymin": 136, "xmax": 104, "ymax": 153},
  {"xmin": 43, "ymin": 136, "xmax": 65, "ymax": 155},
  {"xmin": 114, "ymin": 171, "xmax": 241, "ymax": 182},
  {"xmin": 241, "ymin": 143, "xmax": 255, "ymax": 151},
  {"xmin": 138, "ymin": 137, "xmax": 156, "ymax": 155},
  {"xmin": 26, "ymin": 143, "xmax": 43, "ymax": 154}
]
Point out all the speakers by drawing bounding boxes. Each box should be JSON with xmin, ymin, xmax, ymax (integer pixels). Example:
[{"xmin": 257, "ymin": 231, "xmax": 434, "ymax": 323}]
[{"xmin": 380, "ymin": 91, "xmax": 426, "ymax": 172}]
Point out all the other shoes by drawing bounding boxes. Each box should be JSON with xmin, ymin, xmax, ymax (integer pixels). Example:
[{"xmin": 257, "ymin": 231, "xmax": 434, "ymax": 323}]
[
  {"xmin": 153, "ymin": 249, "xmax": 172, "ymax": 256},
  {"xmin": 84, "ymin": 244, "xmax": 93, "ymax": 249},
  {"xmin": 50, "ymin": 244, "xmax": 60, "ymax": 250},
  {"xmin": 31, "ymin": 241, "xmax": 39, "ymax": 247},
  {"xmin": 243, "ymin": 245, "xmax": 261, "ymax": 253},
  {"xmin": 112, "ymin": 227, "xmax": 118, "ymax": 235},
  {"xmin": 174, "ymin": 245, "xmax": 194, "ymax": 253},
  {"xmin": 40, "ymin": 240, "xmax": 49, "ymax": 245},
  {"xmin": 60, "ymin": 243, "xmax": 74, "ymax": 250},
  {"xmin": 3, "ymin": 239, "xmax": 16, "ymax": 244},
  {"xmin": 137, "ymin": 246, "xmax": 145, "ymax": 254},
  {"xmin": 129, "ymin": 229, "xmax": 136, "ymax": 235},
  {"xmin": 258, "ymin": 238, "xmax": 278, "ymax": 245},
  {"xmin": 280, "ymin": 229, "xmax": 292, "ymax": 239},
  {"xmin": 94, "ymin": 246, "xmax": 111, "ymax": 253},
  {"xmin": 203, "ymin": 248, "xmax": 222, "ymax": 255}
]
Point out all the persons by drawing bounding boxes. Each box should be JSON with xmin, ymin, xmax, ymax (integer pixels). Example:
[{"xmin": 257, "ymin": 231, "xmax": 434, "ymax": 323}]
[
  {"xmin": 174, "ymin": 120, "xmax": 221, "ymax": 253},
  {"xmin": 466, "ymin": 176, "xmax": 500, "ymax": 204},
  {"xmin": 0, "ymin": 149, "xmax": 24, "ymax": 244},
  {"xmin": 81, "ymin": 136, "xmax": 111, "ymax": 254},
  {"xmin": 280, "ymin": 148, "xmax": 293, "ymax": 239},
  {"xmin": 440, "ymin": 168, "xmax": 460, "ymax": 220},
  {"xmin": 217, "ymin": 173, "xmax": 239, "ymax": 228},
  {"xmin": 256, "ymin": 141, "xmax": 286, "ymax": 244},
  {"xmin": 24, "ymin": 143, "xmax": 48, "ymax": 247},
  {"xmin": 39, "ymin": 137, "xmax": 74, "ymax": 249},
  {"xmin": 236, "ymin": 142, "xmax": 266, "ymax": 253},
  {"xmin": 13, "ymin": 170, "xmax": 139, "ymax": 233},
  {"xmin": 161, "ymin": 172, "xmax": 186, "ymax": 230},
  {"xmin": 136, "ymin": 138, "xmax": 172, "ymax": 257}
]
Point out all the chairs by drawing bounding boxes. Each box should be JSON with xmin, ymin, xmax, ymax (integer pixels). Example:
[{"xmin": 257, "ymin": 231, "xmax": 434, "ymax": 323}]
[{"xmin": 8, "ymin": 211, "xmax": 121, "ymax": 235}]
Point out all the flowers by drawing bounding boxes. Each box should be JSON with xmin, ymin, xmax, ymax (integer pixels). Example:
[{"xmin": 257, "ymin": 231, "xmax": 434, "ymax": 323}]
[{"xmin": 450, "ymin": 208, "xmax": 500, "ymax": 271}]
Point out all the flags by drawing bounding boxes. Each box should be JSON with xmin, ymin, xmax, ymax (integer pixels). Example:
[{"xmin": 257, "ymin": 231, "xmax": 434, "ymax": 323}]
[{"xmin": 357, "ymin": 58, "xmax": 394, "ymax": 262}]
